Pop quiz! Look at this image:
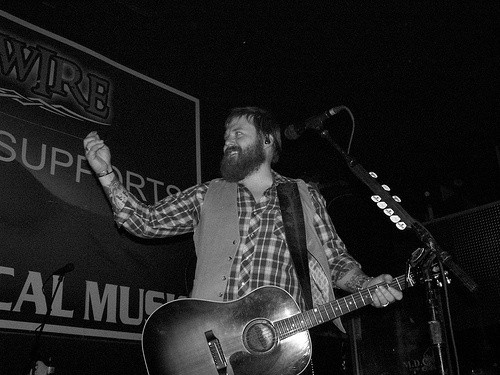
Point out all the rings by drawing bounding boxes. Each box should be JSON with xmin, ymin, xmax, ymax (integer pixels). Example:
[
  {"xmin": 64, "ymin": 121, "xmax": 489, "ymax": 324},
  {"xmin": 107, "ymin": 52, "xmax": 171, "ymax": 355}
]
[
  {"xmin": 382, "ymin": 302, "xmax": 390, "ymax": 307},
  {"xmin": 86, "ymin": 146, "xmax": 91, "ymax": 151}
]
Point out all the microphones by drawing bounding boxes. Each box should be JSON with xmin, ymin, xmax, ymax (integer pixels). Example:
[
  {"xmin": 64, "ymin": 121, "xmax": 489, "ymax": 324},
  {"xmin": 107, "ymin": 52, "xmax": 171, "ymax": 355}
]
[
  {"xmin": 283, "ymin": 107, "xmax": 339, "ymax": 139},
  {"xmin": 51, "ymin": 264, "xmax": 75, "ymax": 276}
]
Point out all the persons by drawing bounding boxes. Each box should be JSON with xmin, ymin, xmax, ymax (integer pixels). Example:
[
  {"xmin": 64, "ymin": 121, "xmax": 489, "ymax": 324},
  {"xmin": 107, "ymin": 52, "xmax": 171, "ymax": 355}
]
[
  {"xmin": 30, "ymin": 361, "xmax": 56, "ymax": 375},
  {"xmin": 84, "ymin": 108, "xmax": 404, "ymax": 375}
]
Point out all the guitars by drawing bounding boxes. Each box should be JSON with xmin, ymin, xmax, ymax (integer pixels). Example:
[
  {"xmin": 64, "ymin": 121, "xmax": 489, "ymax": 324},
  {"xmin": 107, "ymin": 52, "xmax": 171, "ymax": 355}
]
[{"xmin": 140, "ymin": 260, "xmax": 450, "ymax": 375}]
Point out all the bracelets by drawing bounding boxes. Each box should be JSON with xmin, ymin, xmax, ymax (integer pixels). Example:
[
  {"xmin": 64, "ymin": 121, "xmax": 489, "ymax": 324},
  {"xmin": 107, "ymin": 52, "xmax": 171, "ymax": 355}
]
[
  {"xmin": 354, "ymin": 276, "xmax": 373, "ymax": 294},
  {"xmin": 96, "ymin": 168, "xmax": 113, "ymax": 178}
]
